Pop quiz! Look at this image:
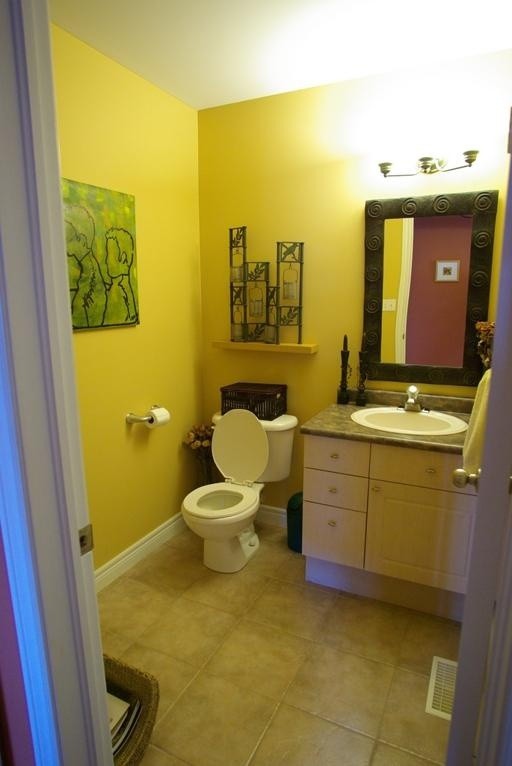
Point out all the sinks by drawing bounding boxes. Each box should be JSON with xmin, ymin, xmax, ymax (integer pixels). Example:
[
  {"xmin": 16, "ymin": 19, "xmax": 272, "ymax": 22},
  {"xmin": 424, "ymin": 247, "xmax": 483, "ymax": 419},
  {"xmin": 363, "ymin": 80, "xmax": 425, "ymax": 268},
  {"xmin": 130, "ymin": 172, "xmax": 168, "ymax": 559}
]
[{"xmin": 350, "ymin": 406, "xmax": 470, "ymax": 436}]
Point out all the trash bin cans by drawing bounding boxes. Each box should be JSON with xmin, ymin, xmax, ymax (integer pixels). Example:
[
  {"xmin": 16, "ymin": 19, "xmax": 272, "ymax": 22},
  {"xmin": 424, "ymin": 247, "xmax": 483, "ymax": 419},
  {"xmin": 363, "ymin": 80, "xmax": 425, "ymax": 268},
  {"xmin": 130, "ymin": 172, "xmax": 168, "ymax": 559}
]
[{"xmin": 285, "ymin": 492, "xmax": 302, "ymax": 553}]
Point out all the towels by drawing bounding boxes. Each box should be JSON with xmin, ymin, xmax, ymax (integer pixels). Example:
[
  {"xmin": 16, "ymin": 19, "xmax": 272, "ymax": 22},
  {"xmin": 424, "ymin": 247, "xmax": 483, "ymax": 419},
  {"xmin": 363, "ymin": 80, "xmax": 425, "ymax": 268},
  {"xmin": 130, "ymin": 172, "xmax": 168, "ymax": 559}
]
[{"xmin": 462, "ymin": 368, "xmax": 493, "ymax": 476}]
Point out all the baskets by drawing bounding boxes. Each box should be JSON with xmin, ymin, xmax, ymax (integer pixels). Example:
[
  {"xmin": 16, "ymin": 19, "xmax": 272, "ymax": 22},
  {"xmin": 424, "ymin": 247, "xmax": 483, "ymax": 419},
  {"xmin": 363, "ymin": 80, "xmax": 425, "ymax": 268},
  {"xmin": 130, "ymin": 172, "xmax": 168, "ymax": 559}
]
[{"xmin": 221, "ymin": 383, "xmax": 287, "ymax": 420}]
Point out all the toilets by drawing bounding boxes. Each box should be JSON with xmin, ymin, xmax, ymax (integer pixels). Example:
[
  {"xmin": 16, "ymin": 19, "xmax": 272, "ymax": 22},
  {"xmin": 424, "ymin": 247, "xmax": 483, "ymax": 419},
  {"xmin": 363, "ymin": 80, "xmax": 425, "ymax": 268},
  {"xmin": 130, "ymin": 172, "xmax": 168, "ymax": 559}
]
[{"xmin": 181, "ymin": 410, "xmax": 298, "ymax": 575}]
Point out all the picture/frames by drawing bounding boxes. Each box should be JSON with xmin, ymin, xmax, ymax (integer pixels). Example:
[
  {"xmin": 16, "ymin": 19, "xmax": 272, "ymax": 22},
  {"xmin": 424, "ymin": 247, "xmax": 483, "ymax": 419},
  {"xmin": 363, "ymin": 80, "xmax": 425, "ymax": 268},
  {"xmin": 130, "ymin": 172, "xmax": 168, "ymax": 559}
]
[{"xmin": 433, "ymin": 259, "xmax": 459, "ymax": 282}]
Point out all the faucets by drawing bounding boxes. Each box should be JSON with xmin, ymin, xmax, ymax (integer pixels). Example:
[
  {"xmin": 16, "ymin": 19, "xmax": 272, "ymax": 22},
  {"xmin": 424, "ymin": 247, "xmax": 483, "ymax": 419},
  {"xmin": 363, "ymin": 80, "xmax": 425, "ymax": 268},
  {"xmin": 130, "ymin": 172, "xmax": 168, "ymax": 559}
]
[{"xmin": 404, "ymin": 384, "xmax": 422, "ymax": 411}]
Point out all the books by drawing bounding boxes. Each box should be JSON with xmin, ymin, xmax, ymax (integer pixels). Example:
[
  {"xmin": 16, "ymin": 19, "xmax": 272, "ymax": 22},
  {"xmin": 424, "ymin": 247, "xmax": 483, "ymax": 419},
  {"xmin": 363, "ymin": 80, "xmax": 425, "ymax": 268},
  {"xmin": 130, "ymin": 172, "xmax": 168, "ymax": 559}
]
[{"xmin": 107, "ymin": 679, "xmax": 144, "ymax": 761}]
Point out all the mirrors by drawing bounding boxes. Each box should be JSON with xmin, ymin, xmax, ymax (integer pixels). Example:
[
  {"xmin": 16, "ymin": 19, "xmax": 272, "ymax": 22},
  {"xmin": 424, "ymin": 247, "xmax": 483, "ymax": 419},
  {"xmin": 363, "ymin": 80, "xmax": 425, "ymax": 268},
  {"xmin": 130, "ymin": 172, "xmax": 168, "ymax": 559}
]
[{"xmin": 360, "ymin": 190, "xmax": 499, "ymax": 388}]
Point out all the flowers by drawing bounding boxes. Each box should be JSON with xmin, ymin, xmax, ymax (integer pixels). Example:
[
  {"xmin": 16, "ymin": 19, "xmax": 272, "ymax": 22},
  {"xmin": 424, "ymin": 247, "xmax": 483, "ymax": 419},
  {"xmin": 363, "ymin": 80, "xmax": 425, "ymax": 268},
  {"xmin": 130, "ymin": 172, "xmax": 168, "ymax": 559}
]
[{"xmin": 181, "ymin": 424, "xmax": 216, "ymax": 482}]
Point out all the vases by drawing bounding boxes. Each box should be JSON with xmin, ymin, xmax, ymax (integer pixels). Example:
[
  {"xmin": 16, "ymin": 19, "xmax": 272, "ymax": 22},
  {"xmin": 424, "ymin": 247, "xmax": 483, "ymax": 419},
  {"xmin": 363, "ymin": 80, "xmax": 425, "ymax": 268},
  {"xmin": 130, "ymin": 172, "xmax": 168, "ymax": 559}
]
[{"xmin": 199, "ymin": 464, "xmax": 211, "ymax": 487}]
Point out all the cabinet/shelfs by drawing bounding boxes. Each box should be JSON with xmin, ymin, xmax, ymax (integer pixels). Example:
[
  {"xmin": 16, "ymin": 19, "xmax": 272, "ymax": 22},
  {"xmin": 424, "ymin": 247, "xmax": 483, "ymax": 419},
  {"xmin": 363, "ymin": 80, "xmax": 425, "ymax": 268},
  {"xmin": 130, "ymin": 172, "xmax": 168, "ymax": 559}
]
[{"xmin": 301, "ymin": 432, "xmax": 478, "ymax": 624}]
